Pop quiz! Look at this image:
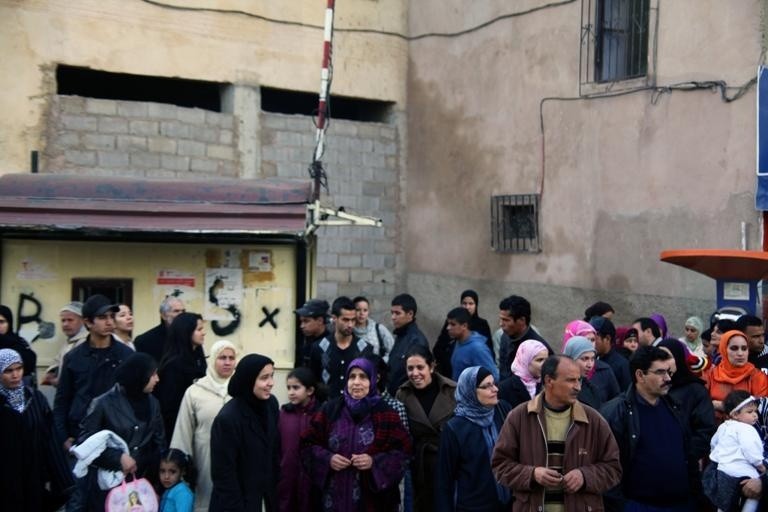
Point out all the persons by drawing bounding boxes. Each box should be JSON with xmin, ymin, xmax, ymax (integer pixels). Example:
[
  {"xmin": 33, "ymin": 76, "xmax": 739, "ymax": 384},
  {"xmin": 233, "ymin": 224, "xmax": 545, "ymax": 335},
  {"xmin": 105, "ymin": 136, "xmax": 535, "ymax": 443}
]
[{"xmin": 2, "ymin": 290, "xmax": 768, "ymax": 511}]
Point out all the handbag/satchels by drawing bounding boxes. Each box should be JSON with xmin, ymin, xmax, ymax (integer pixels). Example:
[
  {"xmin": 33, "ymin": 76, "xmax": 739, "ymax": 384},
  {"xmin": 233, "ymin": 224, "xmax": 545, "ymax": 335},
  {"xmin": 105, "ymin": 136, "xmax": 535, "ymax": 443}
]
[{"xmin": 104, "ymin": 472, "xmax": 160, "ymax": 512}]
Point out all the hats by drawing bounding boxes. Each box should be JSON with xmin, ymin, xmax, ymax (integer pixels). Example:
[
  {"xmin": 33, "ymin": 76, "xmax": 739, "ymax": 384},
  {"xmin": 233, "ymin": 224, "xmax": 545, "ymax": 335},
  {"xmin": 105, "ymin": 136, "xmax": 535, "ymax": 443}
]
[
  {"xmin": 82, "ymin": 293, "xmax": 120, "ymax": 315},
  {"xmin": 687, "ymin": 355, "xmax": 711, "ymax": 377},
  {"xmin": 292, "ymin": 299, "xmax": 328, "ymax": 317}
]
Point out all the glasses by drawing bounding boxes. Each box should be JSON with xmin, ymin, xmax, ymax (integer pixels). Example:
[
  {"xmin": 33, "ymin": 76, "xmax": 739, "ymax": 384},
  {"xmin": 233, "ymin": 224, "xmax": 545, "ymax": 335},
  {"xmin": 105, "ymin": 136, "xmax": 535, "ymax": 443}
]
[
  {"xmin": 646, "ymin": 369, "xmax": 674, "ymax": 376},
  {"xmin": 477, "ymin": 381, "xmax": 495, "ymax": 389}
]
